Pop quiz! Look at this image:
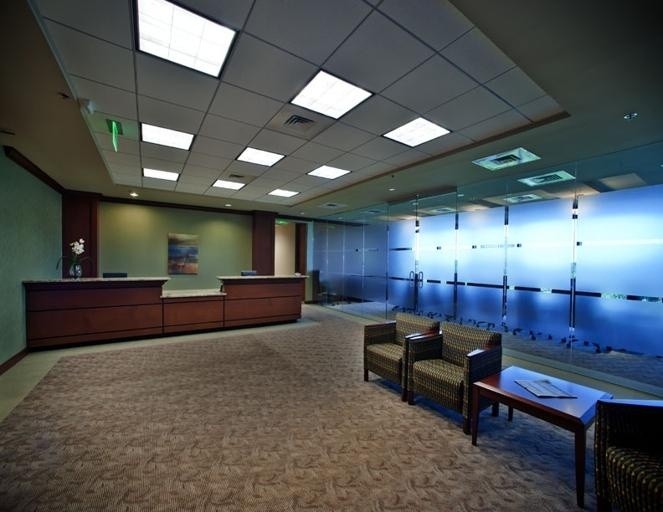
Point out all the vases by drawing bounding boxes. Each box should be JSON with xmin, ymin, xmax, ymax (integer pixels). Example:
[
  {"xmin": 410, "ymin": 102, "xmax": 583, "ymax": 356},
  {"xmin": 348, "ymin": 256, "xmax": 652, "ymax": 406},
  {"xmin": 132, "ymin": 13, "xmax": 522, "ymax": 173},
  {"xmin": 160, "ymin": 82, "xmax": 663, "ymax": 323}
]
[{"xmin": 68, "ymin": 264, "xmax": 81, "ymax": 278}]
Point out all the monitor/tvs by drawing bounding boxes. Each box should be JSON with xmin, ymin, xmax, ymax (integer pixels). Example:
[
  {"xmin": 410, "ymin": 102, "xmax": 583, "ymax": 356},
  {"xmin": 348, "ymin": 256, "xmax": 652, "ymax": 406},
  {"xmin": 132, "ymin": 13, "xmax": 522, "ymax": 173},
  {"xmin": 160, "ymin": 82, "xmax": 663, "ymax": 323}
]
[
  {"xmin": 102, "ymin": 272, "xmax": 128, "ymax": 278},
  {"xmin": 241, "ymin": 271, "xmax": 257, "ymax": 275}
]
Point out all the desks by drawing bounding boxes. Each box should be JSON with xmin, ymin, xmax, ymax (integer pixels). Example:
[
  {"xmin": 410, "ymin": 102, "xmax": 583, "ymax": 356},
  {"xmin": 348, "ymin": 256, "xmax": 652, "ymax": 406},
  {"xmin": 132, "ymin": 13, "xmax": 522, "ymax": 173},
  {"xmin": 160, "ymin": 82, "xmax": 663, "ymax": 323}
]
[{"xmin": 471, "ymin": 363, "xmax": 613, "ymax": 507}]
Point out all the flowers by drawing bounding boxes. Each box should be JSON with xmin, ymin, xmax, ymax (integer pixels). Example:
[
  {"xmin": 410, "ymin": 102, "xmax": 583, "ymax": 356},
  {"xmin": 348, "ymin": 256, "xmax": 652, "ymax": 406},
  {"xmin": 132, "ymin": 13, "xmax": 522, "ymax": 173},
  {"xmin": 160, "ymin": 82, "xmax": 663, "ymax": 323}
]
[{"xmin": 53, "ymin": 237, "xmax": 94, "ymax": 279}]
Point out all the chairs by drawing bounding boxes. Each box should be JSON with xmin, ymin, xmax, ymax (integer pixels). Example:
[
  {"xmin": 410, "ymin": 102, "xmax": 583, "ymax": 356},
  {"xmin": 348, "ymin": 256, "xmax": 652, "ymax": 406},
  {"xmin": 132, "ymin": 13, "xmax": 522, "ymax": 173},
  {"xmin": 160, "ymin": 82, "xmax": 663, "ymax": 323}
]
[
  {"xmin": 358, "ymin": 315, "xmax": 503, "ymax": 436},
  {"xmin": 593, "ymin": 396, "xmax": 663, "ymax": 510}
]
